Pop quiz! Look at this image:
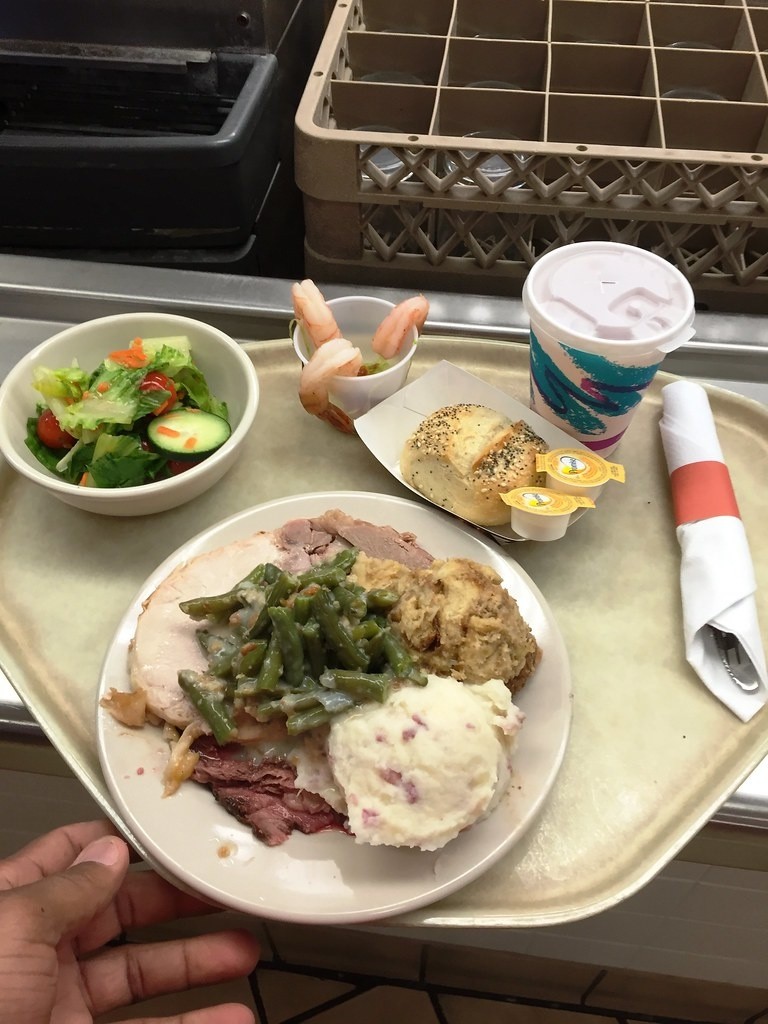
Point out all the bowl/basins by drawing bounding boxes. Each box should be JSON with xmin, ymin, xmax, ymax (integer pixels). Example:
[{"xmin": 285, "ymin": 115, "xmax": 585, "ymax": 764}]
[{"xmin": 0, "ymin": 312, "xmax": 259, "ymax": 517}]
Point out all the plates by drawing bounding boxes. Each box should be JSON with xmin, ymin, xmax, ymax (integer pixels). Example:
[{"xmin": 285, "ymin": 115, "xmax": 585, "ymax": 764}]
[{"xmin": 96, "ymin": 490, "xmax": 571, "ymax": 925}]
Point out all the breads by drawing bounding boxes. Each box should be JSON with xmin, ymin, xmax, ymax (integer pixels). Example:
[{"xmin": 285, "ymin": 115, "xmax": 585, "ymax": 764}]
[{"xmin": 400, "ymin": 402, "xmax": 550, "ymax": 526}]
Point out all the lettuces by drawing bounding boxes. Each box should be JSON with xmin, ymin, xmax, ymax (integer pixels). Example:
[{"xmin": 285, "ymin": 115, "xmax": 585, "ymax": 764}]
[{"xmin": 23, "ymin": 332, "xmax": 229, "ymax": 488}]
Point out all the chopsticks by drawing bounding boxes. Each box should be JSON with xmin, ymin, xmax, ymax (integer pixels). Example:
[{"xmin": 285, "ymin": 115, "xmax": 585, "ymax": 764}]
[{"xmin": 0, "ymin": 82, "xmax": 238, "ymax": 134}]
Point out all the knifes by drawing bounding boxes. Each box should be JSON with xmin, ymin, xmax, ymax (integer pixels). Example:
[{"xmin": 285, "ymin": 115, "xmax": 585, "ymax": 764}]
[{"xmin": 711, "ymin": 628, "xmax": 762, "ymax": 691}]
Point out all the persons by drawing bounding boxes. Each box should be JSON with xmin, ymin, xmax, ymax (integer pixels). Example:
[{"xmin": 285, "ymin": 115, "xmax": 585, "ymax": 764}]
[{"xmin": 0, "ymin": 818, "xmax": 262, "ymax": 1024}]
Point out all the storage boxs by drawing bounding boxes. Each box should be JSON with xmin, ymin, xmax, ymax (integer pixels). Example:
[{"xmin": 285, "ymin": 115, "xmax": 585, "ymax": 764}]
[{"xmin": 294, "ymin": 0, "xmax": 767, "ymax": 319}]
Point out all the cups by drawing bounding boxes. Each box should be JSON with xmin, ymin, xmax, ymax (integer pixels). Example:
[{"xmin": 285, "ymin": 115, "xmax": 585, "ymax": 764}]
[
  {"xmin": 664, "ymin": 86, "xmax": 731, "ymax": 102},
  {"xmin": 293, "ymin": 298, "xmax": 419, "ymax": 434},
  {"xmin": 360, "ymin": 72, "xmax": 523, "ymax": 92},
  {"xmin": 521, "ymin": 241, "xmax": 695, "ymax": 460},
  {"xmin": 339, "ymin": 124, "xmax": 527, "ymax": 189}
]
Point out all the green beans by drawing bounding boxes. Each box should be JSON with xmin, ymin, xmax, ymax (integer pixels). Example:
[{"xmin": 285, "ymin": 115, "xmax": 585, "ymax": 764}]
[{"xmin": 175, "ymin": 551, "xmax": 431, "ymax": 743}]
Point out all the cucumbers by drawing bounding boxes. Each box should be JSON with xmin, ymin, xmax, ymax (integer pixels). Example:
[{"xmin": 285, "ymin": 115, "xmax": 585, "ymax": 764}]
[{"xmin": 145, "ymin": 408, "xmax": 231, "ymax": 462}]
